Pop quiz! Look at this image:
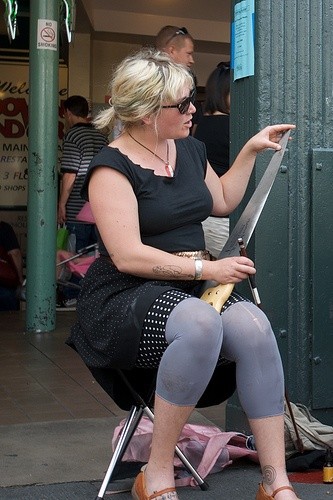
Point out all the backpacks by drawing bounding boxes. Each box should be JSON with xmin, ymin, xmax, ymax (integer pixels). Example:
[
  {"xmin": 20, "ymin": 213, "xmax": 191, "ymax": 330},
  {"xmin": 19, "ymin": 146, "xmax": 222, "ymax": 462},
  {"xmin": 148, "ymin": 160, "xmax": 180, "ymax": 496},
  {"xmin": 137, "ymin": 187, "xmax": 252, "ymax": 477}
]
[
  {"xmin": 105, "ymin": 414, "xmax": 260, "ymax": 494},
  {"xmin": 284, "ymin": 401, "xmax": 333, "ymax": 470}
]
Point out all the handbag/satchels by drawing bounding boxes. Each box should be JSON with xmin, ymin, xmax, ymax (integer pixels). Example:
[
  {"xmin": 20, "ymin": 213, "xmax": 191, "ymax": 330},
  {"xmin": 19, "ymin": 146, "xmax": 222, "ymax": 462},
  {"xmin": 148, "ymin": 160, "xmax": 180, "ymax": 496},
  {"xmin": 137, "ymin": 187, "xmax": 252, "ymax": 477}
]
[
  {"xmin": 56, "ymin": 221, "xmax": 76, "ymax": 282},
  {"xmin": 0, "ymin": 245, "xmax": 20, "ymax": 289}
]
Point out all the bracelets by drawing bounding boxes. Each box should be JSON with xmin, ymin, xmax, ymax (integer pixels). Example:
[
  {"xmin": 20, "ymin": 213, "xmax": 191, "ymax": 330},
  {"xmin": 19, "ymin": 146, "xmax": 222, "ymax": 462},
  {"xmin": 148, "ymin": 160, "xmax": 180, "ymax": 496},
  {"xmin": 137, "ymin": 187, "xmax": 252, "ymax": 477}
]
[{"xmin": 192, "ymin": 258, "xmax": 206, "ymax": 283}]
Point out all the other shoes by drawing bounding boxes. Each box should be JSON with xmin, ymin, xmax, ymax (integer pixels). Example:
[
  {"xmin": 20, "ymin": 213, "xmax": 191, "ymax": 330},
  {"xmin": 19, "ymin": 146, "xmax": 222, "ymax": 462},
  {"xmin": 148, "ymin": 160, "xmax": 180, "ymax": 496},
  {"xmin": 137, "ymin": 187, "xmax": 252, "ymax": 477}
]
[
  {"xmin": 256, "ymin": 480, "xmax": 301, "ymax": 500},
  {"xmin": 131, "ymin": 464, "xmax": 179, "ymax": 500}
]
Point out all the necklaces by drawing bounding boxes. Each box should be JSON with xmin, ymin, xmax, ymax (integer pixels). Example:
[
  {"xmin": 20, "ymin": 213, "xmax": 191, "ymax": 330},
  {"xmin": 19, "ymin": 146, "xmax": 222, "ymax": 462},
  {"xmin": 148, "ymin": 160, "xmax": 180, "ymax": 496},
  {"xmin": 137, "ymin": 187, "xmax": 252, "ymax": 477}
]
[{"xmin": 125, "ymin": 127, "xmax": 175, "ymax": 177}]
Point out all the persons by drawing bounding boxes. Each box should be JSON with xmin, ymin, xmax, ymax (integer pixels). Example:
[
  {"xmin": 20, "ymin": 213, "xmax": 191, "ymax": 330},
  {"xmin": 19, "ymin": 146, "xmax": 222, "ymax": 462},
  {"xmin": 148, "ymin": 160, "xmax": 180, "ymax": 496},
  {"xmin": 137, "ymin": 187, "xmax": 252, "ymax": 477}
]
[
  {"xmin": 191, "ymin": 61, "xmax": 232, "ymax": 176},
  {"xmin": 0, "ymin": 221, "xmax": 24, "ymax": 311},
  {"xmin": 70, "ymin": 48, "xmax": 302, "ymax": 500},
  {"xmin": 151, "ymin": 25, "xmax": 195, "ymax": 72},
  {"xmin": 58, "ymin": 95, "xmax": 109, "ymax": 254}
]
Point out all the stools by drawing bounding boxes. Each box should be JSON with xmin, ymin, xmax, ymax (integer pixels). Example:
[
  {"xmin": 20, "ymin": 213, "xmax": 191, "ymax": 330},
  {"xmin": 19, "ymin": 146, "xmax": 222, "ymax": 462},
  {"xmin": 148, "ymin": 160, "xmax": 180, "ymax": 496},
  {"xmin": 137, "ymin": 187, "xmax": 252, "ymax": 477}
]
[{"xmin": 97, "ymin": 369, "xmax": 209, "ymax": 500}]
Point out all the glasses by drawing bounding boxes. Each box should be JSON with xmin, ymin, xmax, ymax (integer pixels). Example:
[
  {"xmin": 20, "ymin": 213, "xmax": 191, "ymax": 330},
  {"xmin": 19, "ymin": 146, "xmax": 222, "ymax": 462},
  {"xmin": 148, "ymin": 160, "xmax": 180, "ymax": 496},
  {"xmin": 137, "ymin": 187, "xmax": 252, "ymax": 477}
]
[
  {"xmin": 166, "ymin": 27, "xmax": 189, "ymax": 45},
  {"xmin": 156, "ymin": 88, "xmax": 198, "ymax": 114},
  {"xmin": 217, "ymin": 62, "xmax": 230, "ymax": 75}
]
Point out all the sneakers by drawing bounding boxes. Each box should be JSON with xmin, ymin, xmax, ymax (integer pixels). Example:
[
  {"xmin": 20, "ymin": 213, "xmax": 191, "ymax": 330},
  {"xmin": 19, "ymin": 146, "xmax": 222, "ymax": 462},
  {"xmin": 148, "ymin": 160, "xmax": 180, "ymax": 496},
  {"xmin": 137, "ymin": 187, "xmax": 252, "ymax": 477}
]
[{"xmin": 56, "ymin": 297, "xmax": 77, "ymax": 311}]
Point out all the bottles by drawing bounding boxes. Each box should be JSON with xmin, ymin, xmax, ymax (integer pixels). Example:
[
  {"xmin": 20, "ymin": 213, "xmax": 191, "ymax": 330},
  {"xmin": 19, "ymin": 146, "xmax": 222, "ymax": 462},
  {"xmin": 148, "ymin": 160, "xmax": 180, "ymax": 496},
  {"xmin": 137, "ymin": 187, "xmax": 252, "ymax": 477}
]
[{"xmin": 323, "ymin": 448, "xmax": 333, "ymax": 483}]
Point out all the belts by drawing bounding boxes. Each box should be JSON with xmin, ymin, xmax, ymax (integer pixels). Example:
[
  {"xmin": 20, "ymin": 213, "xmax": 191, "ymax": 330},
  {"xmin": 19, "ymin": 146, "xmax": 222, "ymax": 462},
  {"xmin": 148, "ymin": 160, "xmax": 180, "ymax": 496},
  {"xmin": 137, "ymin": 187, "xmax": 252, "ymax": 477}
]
[{"xmin": 98, "ymin": 250, "xmax": 211, "ymax": 266}]
